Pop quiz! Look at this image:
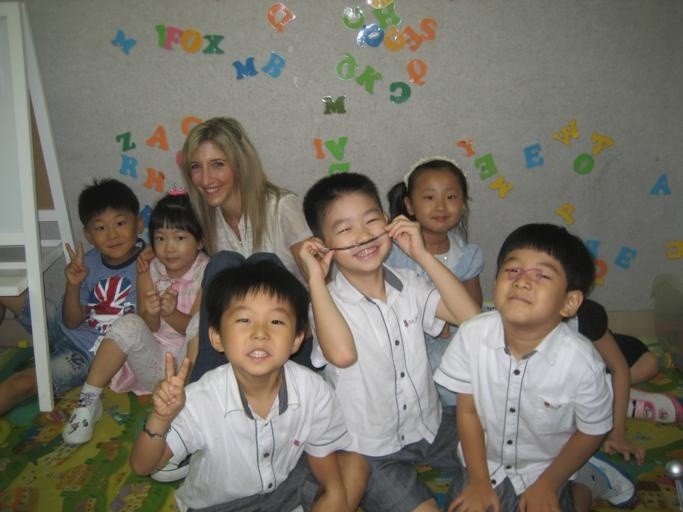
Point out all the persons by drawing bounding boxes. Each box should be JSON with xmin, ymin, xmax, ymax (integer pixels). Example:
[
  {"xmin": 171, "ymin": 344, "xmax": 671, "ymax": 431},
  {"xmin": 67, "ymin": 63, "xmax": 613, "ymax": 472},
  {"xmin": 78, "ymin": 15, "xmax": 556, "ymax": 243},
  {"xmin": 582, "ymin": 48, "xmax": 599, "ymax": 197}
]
[
  {"xmin": 300, "ymin": 173, "xmax": 482, "ymax": 512},
  {"xmin": 561, "ymin": 234, "xmax": 682, "ymax": 424},
  {"xmin": 433, "ymin": 223, "xmax": 637, "ymax": 511},
  {"xmin": 55, "ymin": 118, "xmax": 332, "ymax": 444},
  {"xmin": 130, "ymin": 262, "xmax": 352, "ymax": 511},
  {"xmin": 382, "ymin": 156, "xmax": 490, "ymax": 415}
]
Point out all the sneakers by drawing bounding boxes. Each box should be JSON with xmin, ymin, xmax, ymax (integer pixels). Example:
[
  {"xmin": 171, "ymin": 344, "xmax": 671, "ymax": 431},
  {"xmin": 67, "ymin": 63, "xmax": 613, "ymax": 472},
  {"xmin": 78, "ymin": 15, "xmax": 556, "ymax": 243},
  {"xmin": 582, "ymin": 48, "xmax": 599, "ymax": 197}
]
[
  {"xmin": 588, "ymin": 452, "xmax": 637, "ymax": 509},
  {"xmin": 150, "ymin": 453, "xmax": 192, "ymax": 482},
  {"xmin": 626, "ymin": 386, "xmax": 683, "ymax": 424},
  {"xmin": 432, "ymin": 467, "xmax": 456, "ymax": 481},
  {"xmin": 63, "ymin": 398, "xmax": 103, "ymax": 444}
]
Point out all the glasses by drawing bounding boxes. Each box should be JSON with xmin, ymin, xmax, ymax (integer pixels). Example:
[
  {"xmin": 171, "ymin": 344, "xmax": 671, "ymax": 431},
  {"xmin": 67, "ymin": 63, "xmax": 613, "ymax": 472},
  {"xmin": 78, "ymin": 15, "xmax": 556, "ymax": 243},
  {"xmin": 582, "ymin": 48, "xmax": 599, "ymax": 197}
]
[{"xmin": 509, "ymin": 267, "xmax": 543, "ymax": 282}]
